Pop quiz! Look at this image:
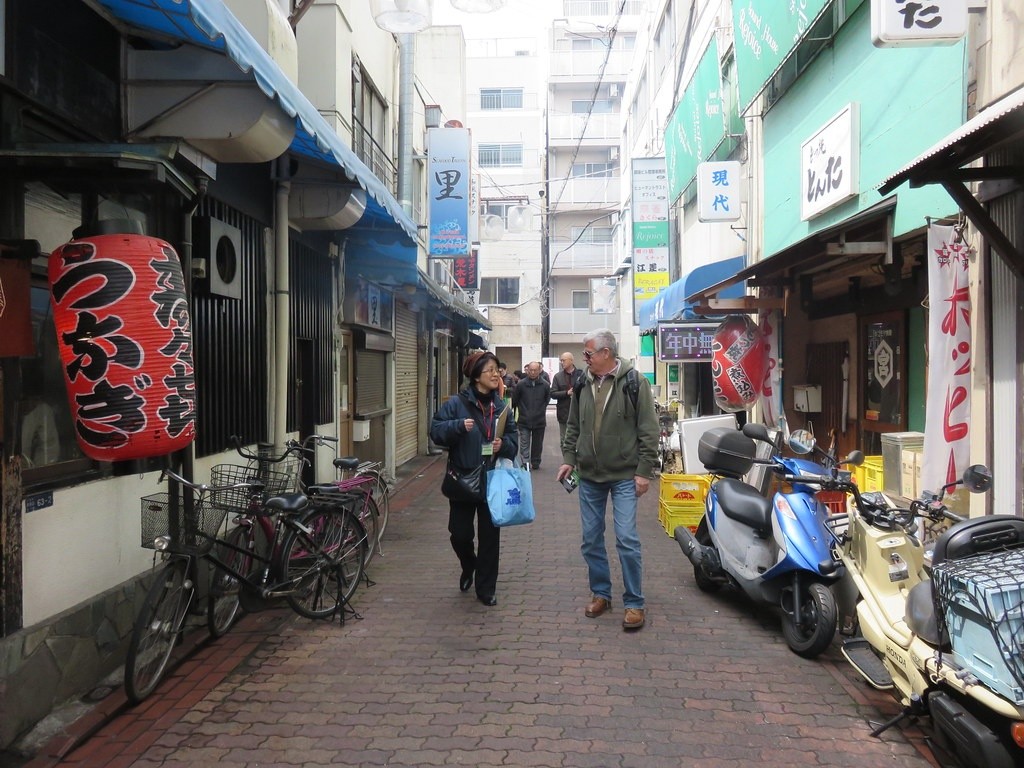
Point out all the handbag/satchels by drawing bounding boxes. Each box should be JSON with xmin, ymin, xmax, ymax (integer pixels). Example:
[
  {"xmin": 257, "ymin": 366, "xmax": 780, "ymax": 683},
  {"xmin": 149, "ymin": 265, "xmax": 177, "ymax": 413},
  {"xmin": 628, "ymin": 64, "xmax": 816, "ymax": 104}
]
[
  {"xmin": 487, "ymin": 454, "xmax": 536, "ymax": 527},
  {"xmin": 442, "ymin": 460, "xmax": 486, "ymax": 502}
]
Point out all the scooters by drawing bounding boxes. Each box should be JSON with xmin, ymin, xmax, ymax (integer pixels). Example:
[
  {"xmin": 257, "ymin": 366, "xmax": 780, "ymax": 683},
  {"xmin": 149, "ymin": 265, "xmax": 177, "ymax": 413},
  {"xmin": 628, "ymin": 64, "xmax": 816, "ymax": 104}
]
[
  {"xmin": 674, "ymin": 423, "xmax": 866, "ymax": 661},
  {"xmin": 782, "ymin": 427, "xmax": 1024, "ymax": 768}
]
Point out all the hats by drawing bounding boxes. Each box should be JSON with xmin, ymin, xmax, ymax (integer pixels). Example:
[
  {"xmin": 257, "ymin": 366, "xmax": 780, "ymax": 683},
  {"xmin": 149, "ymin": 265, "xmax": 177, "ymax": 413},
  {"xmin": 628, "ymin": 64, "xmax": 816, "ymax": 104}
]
[{"xmin": 463, "ymin": 352, "xmax": 494, "ymax": 377}]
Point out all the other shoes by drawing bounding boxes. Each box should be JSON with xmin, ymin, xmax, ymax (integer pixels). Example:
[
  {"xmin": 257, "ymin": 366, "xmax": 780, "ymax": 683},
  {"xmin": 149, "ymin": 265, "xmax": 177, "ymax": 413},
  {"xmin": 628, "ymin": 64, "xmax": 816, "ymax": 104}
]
[
  {"xmin": 531, "ymin": 461, "xmax": 540, "ymax": 469},
  {"xmin": 521, "ymin": 462, "xmax": 530, "ymax": 470}
]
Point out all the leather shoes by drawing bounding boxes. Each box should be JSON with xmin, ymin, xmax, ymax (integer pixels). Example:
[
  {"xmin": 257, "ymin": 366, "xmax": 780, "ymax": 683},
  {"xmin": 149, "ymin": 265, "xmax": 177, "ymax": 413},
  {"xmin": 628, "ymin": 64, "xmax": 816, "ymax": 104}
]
[
  {"xmin": 460, "ymin": 567, "xmax": 474, "ymax": 591},
  {"xmin": 477, "ymin": 594, "xmax": 496, "ymax": 605},
  {"xmin": 585, "ymin": 597, "xmax": 611, "ymax": 618},
  {"xmin": 623, "ymin": 608, "xmax": 645, "ymax": 628}
]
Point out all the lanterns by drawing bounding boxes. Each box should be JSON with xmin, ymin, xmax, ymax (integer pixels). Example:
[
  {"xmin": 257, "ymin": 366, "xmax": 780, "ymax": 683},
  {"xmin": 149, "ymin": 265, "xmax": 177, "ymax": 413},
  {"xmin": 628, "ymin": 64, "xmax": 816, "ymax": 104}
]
[
  {"xmin": 48, "ymin": 219, "xmax": 198, "ymax": 474},
  {"xmin": 711, "ymin": 316, "xmax": 766, "ymax": 411}
]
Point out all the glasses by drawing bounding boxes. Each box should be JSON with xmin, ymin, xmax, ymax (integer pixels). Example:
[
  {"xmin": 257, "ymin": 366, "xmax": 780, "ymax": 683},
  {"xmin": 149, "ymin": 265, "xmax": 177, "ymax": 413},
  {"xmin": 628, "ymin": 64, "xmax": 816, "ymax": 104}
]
[
  {"xmin": 582, "ymin": 349, "xmax": 602, "ymax": 359},
  {"xmin": 482, "ymin": 368, "xmax": 499, "ymax": 373}
]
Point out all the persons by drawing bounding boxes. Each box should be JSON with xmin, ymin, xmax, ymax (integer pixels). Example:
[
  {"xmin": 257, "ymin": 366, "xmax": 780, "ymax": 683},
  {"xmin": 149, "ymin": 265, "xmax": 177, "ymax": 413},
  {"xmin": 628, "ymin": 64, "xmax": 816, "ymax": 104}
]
[
  {"xmin": 429, "ymin": 352, "xmax": 518, "ymax": 605},
  {"xmin": 556, "ymin": 329, "xmax": 659, "ymax": 628},
  {"xmin": 511, "ymin": 362, "xmax": 551, "ymax": 469},
  {"xmin": 500, "ymin": 362, "xmax": 550, "ymax": 418},
  {"xmin": 550, "ymin": 352, "xmax": 583, "ymax": 457}
]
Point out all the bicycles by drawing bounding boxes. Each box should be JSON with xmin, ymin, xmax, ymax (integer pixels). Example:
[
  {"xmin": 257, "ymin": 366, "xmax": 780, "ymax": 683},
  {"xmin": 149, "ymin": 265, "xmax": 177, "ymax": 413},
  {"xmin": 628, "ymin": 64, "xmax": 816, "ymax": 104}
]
[
  {"xmin": 119, "ymin": 450, "xmax": 370, "ymax": 708},
  {"xmin": 203, "ymin": 434, "xmax": 396, "ymax": 641}
]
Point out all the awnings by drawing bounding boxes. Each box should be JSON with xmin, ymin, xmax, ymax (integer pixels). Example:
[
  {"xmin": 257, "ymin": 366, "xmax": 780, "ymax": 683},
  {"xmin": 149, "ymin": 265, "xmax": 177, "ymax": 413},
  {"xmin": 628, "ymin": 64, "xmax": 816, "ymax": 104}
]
[
  {"xmin": 86, "ymin": 0, "xmax": 421, "ymax": 246},
  {"xmin": 684, "ymin": 201, "xmax": 894, "ymax": 314},
  {"xmin": 415, "ymin": 265, "xmax": 492, "ymax": 332},
  {"xmin": 876, "ymin": 86, "xmax": 1024, "ymax": 281},
  {"xmin": 637, "ymin": 257, "xmax": 749, "ymax": 333}
]
